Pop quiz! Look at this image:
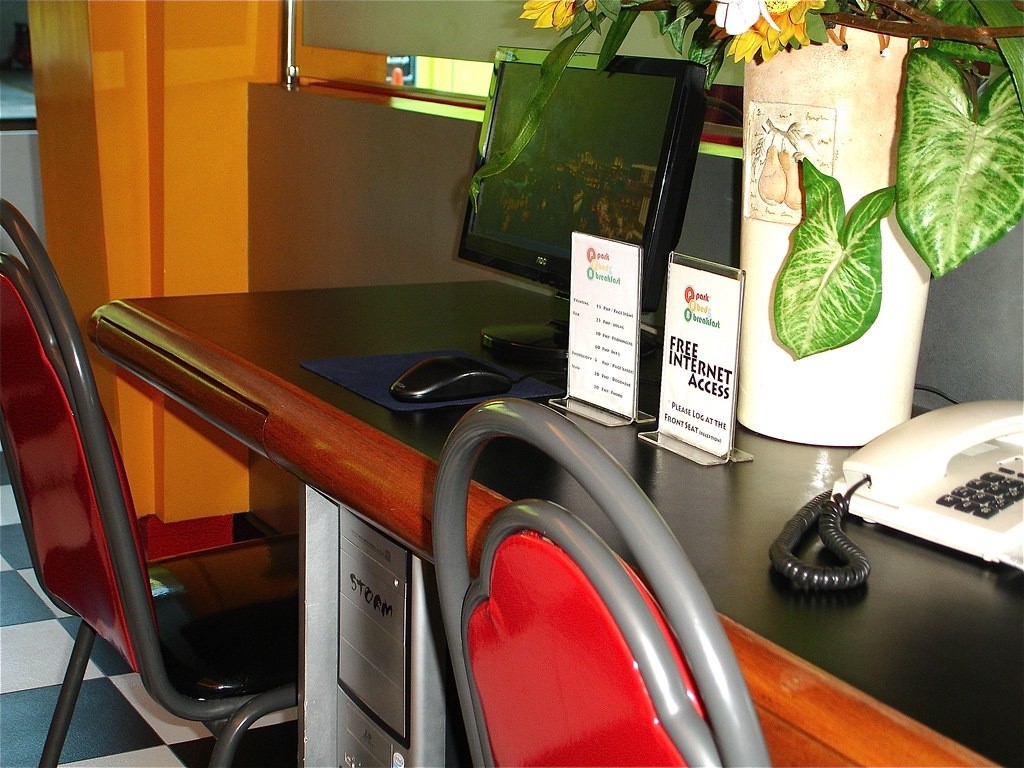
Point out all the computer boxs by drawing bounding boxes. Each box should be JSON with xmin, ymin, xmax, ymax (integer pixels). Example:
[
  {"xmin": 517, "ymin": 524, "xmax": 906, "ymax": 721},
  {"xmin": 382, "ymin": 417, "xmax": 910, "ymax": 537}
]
[{"xmin": 336, "ymin": 504, "xmax": 474, "ymax": 768}]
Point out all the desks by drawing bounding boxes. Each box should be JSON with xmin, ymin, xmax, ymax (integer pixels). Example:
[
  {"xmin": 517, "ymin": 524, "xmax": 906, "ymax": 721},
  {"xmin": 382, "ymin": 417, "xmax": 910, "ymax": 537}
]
[{"xmin": 85, "ymin": 279, "xmax": 1024, "ymax": 768}]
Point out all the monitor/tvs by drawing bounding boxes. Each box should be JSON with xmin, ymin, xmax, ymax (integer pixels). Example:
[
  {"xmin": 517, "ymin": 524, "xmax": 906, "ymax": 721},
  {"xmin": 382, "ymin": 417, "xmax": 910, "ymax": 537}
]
[{"xmin": 457, "ymin": 46, "xmax": 709, "ymax": 361}]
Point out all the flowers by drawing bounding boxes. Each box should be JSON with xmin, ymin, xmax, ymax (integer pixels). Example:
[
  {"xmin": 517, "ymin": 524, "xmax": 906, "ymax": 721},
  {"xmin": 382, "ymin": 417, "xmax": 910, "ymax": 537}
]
[{"xmin": 467, "ymin": 0, "xmax": 1024, "ymax": 359}]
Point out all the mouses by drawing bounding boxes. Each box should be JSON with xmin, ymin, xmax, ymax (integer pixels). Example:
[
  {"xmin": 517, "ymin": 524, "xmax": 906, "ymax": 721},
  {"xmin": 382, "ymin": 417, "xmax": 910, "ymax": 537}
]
[{"xmin": 389, "ymin": 357, "xmax": 513, "ymax": 404}]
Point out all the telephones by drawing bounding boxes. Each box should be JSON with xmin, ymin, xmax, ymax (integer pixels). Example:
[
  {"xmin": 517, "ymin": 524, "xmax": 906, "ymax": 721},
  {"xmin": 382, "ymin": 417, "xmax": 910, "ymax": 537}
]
[{"xmin": 831, "ymin": 400, "xmax": 1023, "ymax": 572}]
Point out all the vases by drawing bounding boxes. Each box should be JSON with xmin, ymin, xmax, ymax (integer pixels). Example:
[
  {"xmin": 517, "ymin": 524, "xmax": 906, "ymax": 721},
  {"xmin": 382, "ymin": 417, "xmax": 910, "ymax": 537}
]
[{"xmin": 733, "ymin": 18, "xmax": 947, "ymax": 442}]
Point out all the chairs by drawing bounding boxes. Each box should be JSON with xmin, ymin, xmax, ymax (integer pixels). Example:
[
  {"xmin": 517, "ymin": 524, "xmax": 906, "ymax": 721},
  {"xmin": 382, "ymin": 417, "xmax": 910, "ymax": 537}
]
[
  {"xmin": 433, "ymin": 401, "xmax": 772, "ymax": 768},
  {"xmin": 1, "ymin": 200, "xmax": 300, "ymax": 767}
]
